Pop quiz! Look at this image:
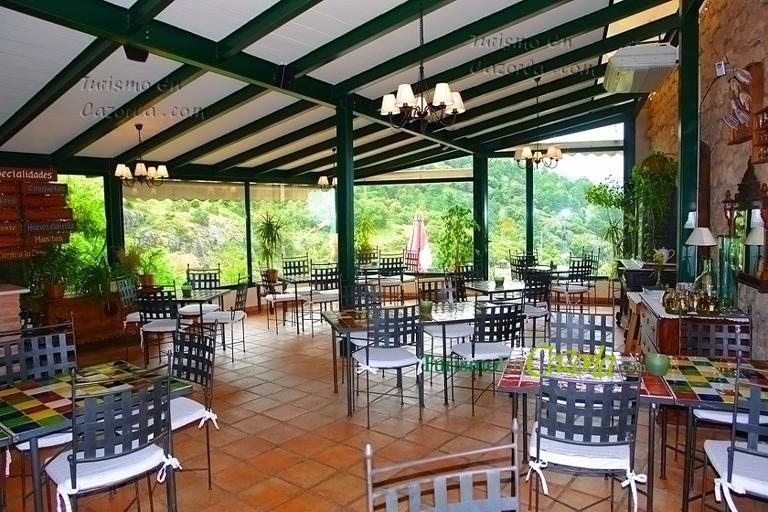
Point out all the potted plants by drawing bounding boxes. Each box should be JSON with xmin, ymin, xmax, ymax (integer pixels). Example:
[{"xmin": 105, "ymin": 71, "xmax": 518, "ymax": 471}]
[{"xmin": 435, "ymin": 203, "xmax": 492, "ymax": 302}]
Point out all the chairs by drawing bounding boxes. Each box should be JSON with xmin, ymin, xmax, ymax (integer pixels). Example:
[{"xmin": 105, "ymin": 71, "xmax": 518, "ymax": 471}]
[{"xmin": 0, "ymin": 248, "xmax": 768, "ymax": 511}]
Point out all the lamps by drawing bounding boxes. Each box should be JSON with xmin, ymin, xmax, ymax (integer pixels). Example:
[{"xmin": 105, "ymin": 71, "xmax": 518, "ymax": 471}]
[
  {"xmin": 380, "ymin": 0, "xmax": 465, "ymax": 133},
  {"xmin": 317, "ymin": 148, "xmax": 337, "ymax": 192},
  {"xmin": 514, "ymin": 77, "xmax": 562, "ymax": 169},
  {"xmin": 684, "ymin": 227, "xmax": 717, "ymax": 283},
  {"xmin": 114, "ymin": 124, "xmax": 170, "ymax": 187},
  {"xmin": 742, "ymin": 227, "xmax": 766, "ymax": 261}
]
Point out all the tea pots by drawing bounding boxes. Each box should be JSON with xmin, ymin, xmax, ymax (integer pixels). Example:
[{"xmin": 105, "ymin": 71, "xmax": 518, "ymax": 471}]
[{"xmin": 653, "ymin": 246, "xmax": 675, "ymax": 263}]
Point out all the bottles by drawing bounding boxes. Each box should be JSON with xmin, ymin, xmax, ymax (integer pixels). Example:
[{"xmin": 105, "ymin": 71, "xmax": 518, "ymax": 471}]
[{"xmin": 700, "ymin": 259, "xmax": 717, "ymax": 298}]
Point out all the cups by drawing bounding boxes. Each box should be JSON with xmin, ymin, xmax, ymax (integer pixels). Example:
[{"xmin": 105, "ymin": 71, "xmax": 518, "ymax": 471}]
[
  {"xmin": 494, "ymin": 276, "xmax": 504, "ymax": 284},
  {"xmin": 646, "ymin": 350, "xmax": 670, "ymax": 378},
  {"xmin": 181, "ymin": 286, "xmax": 192, "ymax": 299},
  {"xmin": 420, "ymin": 302, "xmax": 432, "ymax": 313},
  {"xmin": 420, "ymin": 314, "xmax": 432, "ymax": 321},
  {"xmin": 496, "ymin": 285, "xmax": 504, "ymax": 290}
]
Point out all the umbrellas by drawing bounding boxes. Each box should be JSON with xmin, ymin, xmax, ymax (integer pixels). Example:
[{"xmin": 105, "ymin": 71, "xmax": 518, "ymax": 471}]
[{"xmin": 407, "ymin": 209, "xmax": 432, "ymax": 273}]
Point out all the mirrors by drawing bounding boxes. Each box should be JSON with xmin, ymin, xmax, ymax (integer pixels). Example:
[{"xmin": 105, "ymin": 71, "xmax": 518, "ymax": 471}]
[{"xmin": 721, "ymin": 155, "xmax": 768, "ymax": 294}]
